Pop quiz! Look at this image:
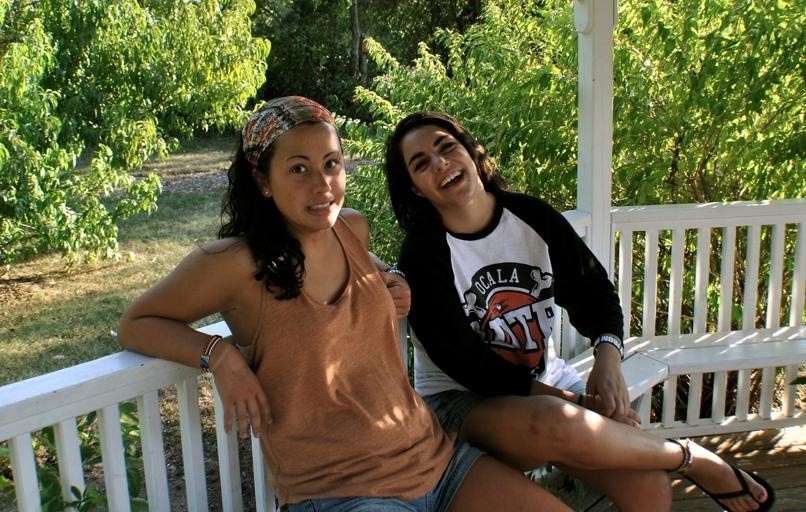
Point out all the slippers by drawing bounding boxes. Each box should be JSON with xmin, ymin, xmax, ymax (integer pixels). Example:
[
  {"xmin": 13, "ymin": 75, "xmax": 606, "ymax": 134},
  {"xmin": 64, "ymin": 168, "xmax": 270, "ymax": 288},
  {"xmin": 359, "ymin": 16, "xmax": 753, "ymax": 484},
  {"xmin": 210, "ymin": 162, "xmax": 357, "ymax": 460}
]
[{"xmin": 682, "ymin": 448, "xmax": 774, "ymax": 511}]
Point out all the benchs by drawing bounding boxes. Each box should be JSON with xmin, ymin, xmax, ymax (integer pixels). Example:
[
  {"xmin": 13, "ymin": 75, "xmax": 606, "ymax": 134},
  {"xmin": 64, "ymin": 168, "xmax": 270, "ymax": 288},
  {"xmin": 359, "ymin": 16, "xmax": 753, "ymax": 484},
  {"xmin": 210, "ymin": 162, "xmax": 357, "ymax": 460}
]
[{"xmin": 567, "ymin": 325, "xmax": 806, "ymax": 403}]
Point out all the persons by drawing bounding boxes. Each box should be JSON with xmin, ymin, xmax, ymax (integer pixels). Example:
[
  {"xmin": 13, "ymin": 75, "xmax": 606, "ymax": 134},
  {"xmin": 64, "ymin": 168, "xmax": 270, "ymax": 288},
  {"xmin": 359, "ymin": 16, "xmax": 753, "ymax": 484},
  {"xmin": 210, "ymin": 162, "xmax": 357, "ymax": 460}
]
[
  {"xmin": 383, "ymin": 109, "xmax": 774, "ymax": 510},
  {"xmin": 115, "ymin": 92, "xmax": 576, "ymax": 511}
]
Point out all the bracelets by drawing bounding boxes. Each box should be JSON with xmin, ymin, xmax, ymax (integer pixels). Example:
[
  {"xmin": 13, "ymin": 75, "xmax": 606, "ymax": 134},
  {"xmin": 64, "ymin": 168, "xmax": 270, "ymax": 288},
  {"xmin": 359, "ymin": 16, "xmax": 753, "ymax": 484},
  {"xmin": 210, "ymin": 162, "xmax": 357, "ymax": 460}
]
[
  {"xmin": 576, "ymin": 394, "xmax": 583, "ymax": 405},
  {"xmin": 198, "ymin": 333, "xmax": 221, "ymax": 372},
  {"xmin": 386, "ymin": 267, "xmax": 406, "ymax": 277},
  {"xmin": 593, "ymin": 335, "xmax": 624, "ymax": 360}
]
